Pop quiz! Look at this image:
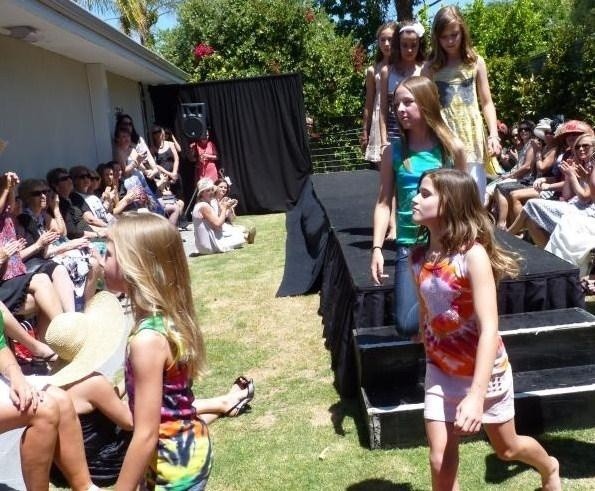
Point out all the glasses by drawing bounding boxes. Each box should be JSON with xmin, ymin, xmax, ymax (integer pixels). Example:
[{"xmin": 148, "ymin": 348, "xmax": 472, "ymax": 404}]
[
  {"xmin": 25, "ymin": 189, "xmax": 49, "ymax": 197},
  {"xmin": 518, "ymin": 127, "xmax": 530, "ymax": 132},
  {"xmin": 75, "ymin": 173, "xmax": 91, "ymax": 180},
  {"xmin": 90, "ymin": 176, "xmax": 100, "ymax": 180},
  {"xmin": 152, "ymin": 129, "xmax": 161, "ymax": 135},
  {"xmin": 545, "ymin": 131, "xmax": 553, "ymax": 135},
  {"xmin": 54, "ymin": 174, "xmax": 71, "ymax": 183}
]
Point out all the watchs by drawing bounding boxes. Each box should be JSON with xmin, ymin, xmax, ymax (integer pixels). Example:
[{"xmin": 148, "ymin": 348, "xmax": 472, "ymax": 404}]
[
  {"xmin": 361, "ymin": 138, "xmax": 369, "ymax": 141},
  {"xmin": 380, "ymin": 144, "xmax": 389, "ymax": 147},
  {"xmin": 371, "ymin": 246, "xmax": 383, "ymax": 250}
]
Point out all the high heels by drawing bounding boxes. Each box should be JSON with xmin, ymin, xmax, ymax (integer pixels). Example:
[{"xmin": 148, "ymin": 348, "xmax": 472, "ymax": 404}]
[
  {"xmin": 222, "ymin": 378, "xmax": 256, "ymax": 419},
  {"xmin": 233, "ymin": 374, "xmax": 251, "ymax": 391}
]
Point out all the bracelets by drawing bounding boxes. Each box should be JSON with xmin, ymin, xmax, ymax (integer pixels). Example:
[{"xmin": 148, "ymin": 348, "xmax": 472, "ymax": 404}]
[{"xmin": 488, "ymin": 136, "xmax": 501, "ymax": 141}]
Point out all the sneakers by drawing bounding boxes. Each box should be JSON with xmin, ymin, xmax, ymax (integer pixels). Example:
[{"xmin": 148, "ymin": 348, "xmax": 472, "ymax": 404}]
[{"xmin": 248, "ymin": 226, "xmax": 257, "ymax": 245}]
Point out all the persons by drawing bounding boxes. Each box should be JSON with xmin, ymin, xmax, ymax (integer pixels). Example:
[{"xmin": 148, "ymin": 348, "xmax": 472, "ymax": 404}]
[
  {"xmin": 154, "ymin": 174, "xmax": 185, "ymax": 226},
  {"xmin": 575, "ymin": 154, "xmax": 595, "ymax": 199},
  {"xmin": 96, "ymin": 163, "xmax": 137, "ymax": 215},
  {"xmin": 412, "ymin": 168, "xmax": 562, "ymax": 490},
  {"xmin": 0, "ymin": 238, "xmax": 60, "ymax": 367},
  {"xmin": 88, "ymin": 169, "xmax": 115, "ymax": 212},
  {"xmin": 214, "ymin": 176, "xmax": 256, "ymax": 244},
  {"xmin": 527, "ymin": 121, "xmax": 594, "ymax": 244},
  {"xmin": 113, "ymin": 127, "xmax": 187, "ymax": 242},
  {"xmin": 370, "ymin": 76, "xmax": 528, "ymax": 339},
  {"xmin": 43, "ymin": 184, "xmax": 68, "ymax": 246},
  {"xmin": 380, "ymin": 21, "xmax": 426, "ymax": 159},
  {"xmin": 118, "ymin": 114, "xmax": 158, "ymax": 194},
  {"xmin": 192, "ymin": 178, "xmax": 245, "ymax": 255},
  {"xmin": 485, "ymin": 119, "xmax": 558, "ymax": 231},
  {"xmin": 46, "ymin": 290, "xmax": 254, "ymax": 488},
  {"xmin": 70, "ymin": 165, "xmax": 110, "ymax": 231},
  {"xmin": 361, "ymin": 20, "xmax": 402, "ymax": 242},
  {"xmin": 187, "ymin": 130, "xmax": 219, "ymax": 186},
  {"xmin": 0, "ymin": 170, "xmax": 74, "ymax": 320},
  {"xmin": 420, "ymin": 4, "xmax": 501, "ymax": 222},
  {"xmin": 12, "ymin": 190, "xmax": 60, "ymax": 263},
  {"xmin": 504, "ymin": 135, "xmax": 595, "ymax": 277},
  {"xmin": 47, "ymin": 168, "xmax": 108, "ymax": 242},
  {"xmin": 148, "ymin": 125, "xmax": 186, "ymax": 231},
  {"xmin": 99, "ymin": 212, "xmax": 212, "ymax": 491},
  {"xmin": 0, "ymin": 308, "xmax": 111, "ymax": 491},
  {"xmin": 108, "ymin": 161, "xmax": 138, "ymax": 210},
  {"xmin": 165, "ymin": 127, "xmax": 182, "ymax": 175},
  {"xmin": 16, "ymin": 178, "xmax": 98, "ymax": 311}
]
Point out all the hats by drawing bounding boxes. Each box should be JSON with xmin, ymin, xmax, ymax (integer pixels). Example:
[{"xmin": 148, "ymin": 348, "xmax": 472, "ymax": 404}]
[
  {"xmin": 536, "ymin": 118, "xmax": 552, "ymax": 132},
  {"xmin": 553, "ymin": 120, "xmax": 585, "ymax": 140},
  {"xmin": 44, "ymin": 291, "xmax": 126, "ymax": 387},
  {"xmin": 197, "ymin": 177, "xmax": 218, "ymax": 198}
]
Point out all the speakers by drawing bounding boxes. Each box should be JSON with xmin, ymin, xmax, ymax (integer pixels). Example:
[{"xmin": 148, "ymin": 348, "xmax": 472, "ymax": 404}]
[{"xmin": 180, "ymin": 103, "xmax": 208, "ymax": 139}]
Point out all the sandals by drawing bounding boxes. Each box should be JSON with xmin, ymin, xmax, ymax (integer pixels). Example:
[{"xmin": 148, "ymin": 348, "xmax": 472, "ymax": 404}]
[{"xmin": 30, "ymin": 352, "xmax": 59, "ymax": 365}]
